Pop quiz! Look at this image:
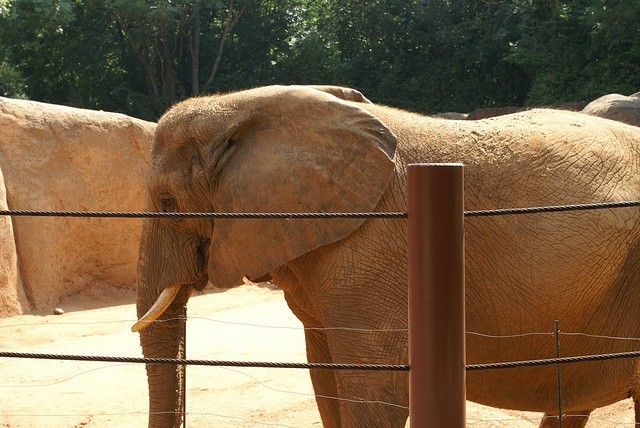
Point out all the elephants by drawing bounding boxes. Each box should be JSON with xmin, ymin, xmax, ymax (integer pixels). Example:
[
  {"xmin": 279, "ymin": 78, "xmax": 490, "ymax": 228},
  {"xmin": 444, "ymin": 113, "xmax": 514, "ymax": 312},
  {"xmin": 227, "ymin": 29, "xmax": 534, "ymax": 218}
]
[{"xmin": 131, "ymin": 83, "xmax": 640, "ymax": 428}]
